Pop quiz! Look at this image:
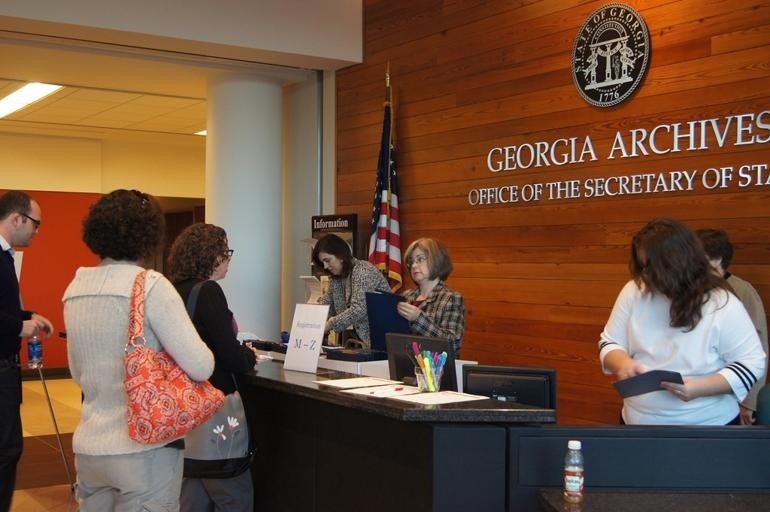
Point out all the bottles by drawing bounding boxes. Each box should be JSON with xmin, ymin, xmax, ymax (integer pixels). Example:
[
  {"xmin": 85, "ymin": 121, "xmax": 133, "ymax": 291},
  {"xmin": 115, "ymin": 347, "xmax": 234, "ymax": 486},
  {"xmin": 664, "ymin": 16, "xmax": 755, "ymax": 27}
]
[
  {"xmin": 26, "ymin": 330, "xmax": 43, "ymax": 370},
  {"xmin": 561, "ymin": 438, "xmax": 584, "ymax": 505}
]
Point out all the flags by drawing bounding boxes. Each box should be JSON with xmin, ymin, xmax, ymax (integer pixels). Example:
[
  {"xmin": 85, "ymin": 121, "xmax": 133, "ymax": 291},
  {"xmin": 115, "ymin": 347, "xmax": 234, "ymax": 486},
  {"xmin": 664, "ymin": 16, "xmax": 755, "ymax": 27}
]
[{"xmin": 364, "ymin": 101, "xmax": 404, "ymax": 296}]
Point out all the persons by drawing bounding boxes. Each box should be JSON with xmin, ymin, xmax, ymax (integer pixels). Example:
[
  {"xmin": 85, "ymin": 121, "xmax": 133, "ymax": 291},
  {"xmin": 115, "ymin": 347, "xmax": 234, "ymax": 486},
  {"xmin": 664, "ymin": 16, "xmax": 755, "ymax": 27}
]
[
  {"xmin": 378, "ymin": 239, "xmax": 467, "ymax": 365},
  {"xmin": 595, "ymin": 218, "xmax": 765, "ymax": 424},
  {"xmin": 304, "ymin": 231, "xmax": 391, "ymax": 352},
  {"xmin": 695, "ymin": 227, "xmax": 770, "ymax": 428},
  {"xmin": 163, "ymin": 221, "xmax": 260, "ymax": 512},
  {"xmin": 0, "ymin": 185, "xmax": 56, "ymax": 511},
  {"xmin": 61, "ymin": 187, "xmax": 215, "ymax": 512}
]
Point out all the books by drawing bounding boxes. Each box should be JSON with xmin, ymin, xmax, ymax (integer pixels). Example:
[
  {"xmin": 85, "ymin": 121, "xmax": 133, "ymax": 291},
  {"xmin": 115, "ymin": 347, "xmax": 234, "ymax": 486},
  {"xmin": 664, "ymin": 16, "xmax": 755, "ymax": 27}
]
[{"xmin": 365, "ymin": 288, "xmax": 411, "ymax": 356}]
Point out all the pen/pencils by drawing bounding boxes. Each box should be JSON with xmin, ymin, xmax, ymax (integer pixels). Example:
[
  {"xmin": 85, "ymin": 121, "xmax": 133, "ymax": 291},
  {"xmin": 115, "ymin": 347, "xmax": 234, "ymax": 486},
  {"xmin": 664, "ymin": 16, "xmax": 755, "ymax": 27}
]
[
  {"xmin": 412, "ymin": 341, "xmax": 448, "ymax": 392},
  {"xmin": 370, "ymin": 387, "xmax": 403, "ymax": 395},
  {"xmin": 272, "ymin": 358, "xmax": 285, "ymax": 363}
]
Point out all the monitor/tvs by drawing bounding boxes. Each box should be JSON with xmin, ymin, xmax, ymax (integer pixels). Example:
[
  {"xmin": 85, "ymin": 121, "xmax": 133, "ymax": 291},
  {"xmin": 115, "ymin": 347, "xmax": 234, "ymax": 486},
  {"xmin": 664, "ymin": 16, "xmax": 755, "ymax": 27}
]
[
  {"xmin": 386, "ymin": 332, "xmax": 458, "ymax": 391},
  {"xmin": 463, "ymin": 364, "xmax": 556, "ymax": 409}
]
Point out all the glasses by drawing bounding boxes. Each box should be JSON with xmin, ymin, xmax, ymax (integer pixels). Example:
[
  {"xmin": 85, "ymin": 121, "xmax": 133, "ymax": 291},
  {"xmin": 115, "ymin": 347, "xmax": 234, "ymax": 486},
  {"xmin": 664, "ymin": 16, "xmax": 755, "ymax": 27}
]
[
  {"xmin": 130, "ymin": 189, "xmax": 151, "ymax": 216},
  {"xmin": 408, "ymin": 255, "xmax": 428, "ymax": 268},
  {"xmin": 220, "ymin": 249, "xmax": 234, "ymax": 255},
  {"xmin": 18, "ymin": 210, "xmax": 40, "ymax": 228}
]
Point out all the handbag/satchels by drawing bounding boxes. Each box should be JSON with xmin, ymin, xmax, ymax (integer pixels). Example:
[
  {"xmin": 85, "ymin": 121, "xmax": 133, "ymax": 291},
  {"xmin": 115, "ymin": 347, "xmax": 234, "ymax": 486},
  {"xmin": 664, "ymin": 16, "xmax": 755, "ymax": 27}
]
[
  {"xmin": 184, "ymin": 390, "xmax": 257, "ymax": 479},
  {"xmin": 123, "ymin": 345, "xmax": 226, "ymax": 445}
]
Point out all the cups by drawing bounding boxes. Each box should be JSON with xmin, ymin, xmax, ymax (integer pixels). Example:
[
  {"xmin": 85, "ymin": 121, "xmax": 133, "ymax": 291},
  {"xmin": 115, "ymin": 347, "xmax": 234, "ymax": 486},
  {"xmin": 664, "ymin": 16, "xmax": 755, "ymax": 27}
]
[{"xmin": 414, "ymin": 366, "xmax": 444, "ymax": 393}]
